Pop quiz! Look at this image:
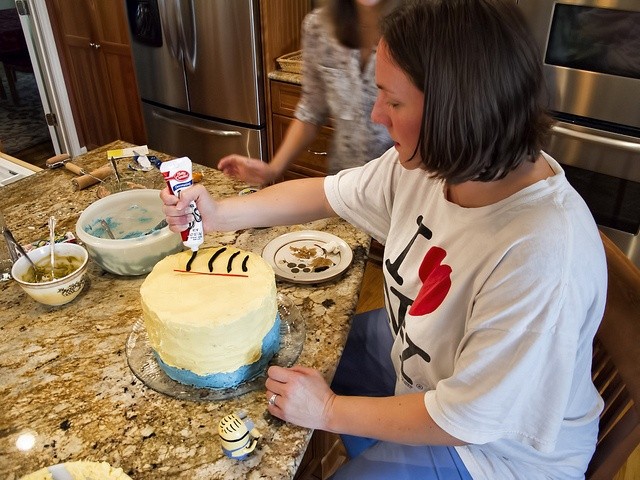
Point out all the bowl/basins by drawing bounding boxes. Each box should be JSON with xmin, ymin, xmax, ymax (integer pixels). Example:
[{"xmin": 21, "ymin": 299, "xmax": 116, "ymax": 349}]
[
  {"xmin": 11, "ymin": 242, "xmax": 88, "ymax": 306},
  {"xmin": 74, "ymin": 188, "xmax": 186, "ymax": 277}
]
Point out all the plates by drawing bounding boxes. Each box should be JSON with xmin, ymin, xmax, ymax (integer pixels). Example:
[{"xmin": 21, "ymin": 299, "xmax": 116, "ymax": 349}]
[
  {"xmin": 95, "ymin": 180, "xmax": 146, "ymax": 200},
  {"xmin": 261, "ymin": 228, "xmax": 353, "ymax": 286}
]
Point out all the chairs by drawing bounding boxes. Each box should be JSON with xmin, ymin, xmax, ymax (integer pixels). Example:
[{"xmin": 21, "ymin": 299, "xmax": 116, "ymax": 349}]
[{"xmin": 586, "ymin": 228, "xmax": 640, "ymax": 480}]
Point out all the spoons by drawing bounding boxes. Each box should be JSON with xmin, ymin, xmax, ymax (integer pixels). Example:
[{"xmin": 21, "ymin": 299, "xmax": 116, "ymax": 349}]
[
  {"xmin": 3, "ymin": 228, "xmax": 48, "ymax": 282},
  {"xmin": 46, "ymin": 215, "xmax": 62, "ymax": 279}
]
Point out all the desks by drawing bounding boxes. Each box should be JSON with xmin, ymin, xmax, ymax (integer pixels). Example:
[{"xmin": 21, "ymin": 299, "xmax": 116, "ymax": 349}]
[{"xmin": 1, "ymin": 138, "xmax": 373, "ymax": 479}]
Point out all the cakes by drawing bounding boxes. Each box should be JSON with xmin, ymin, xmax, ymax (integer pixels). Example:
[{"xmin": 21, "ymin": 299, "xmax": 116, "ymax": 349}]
[{"xmin": 140, "ymin": 247, "xmax": 281, "ymax": 389}]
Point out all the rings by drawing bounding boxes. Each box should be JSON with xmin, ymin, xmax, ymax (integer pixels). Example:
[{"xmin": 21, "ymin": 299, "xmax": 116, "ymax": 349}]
[{"xmin": 269, "ymin": 394, "xmax": 277, "ymax": 406}]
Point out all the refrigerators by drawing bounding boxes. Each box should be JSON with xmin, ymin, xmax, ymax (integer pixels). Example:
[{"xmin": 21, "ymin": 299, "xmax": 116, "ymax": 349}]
[{"xmin": 124, "ymin": 0, "xmax": 268, "ymax": 170}]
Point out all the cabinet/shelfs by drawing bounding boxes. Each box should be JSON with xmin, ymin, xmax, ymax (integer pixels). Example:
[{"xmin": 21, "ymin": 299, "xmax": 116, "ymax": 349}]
[
  {"xmin": 46, "ymin": 0, "xmax": 147, "ymax": 150},
  {"xmin": 267, "ymin": 77, "xmax": 335, "ymax": 183}
]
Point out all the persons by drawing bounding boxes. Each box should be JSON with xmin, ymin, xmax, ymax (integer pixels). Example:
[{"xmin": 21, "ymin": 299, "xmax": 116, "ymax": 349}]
[
  {"xmin": 218, "ymin": 0, "xmax": 405, "ymax": 188},
  {"xmin": 160, "ymin": 0, "xmax": 604, "ymax": 480}
]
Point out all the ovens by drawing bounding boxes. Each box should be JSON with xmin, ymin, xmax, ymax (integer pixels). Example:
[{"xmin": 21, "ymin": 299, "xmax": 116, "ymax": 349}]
[{"xmin": 513, "ymin": 0, "xmax": 640, "ymax": 268}]
